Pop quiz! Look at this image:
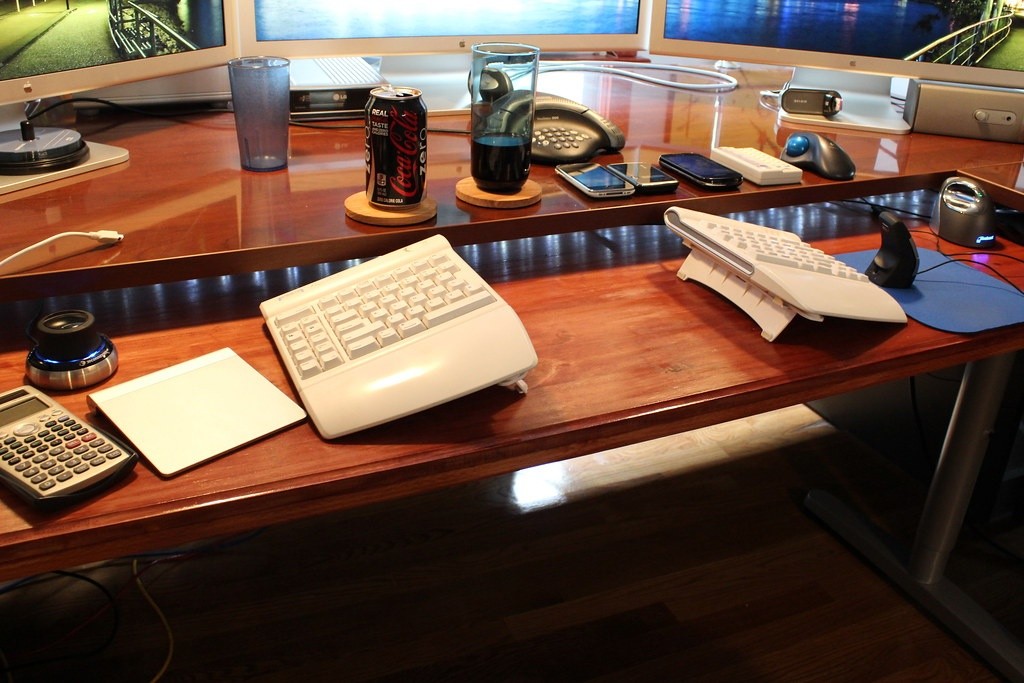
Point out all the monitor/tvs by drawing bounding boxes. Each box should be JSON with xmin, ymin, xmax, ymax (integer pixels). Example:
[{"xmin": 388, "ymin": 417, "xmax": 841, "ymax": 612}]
[
  {"xmin": 0, "ymin": 0, "xmax": 239, "ymax": 109},
  {"xmin": 238, "ymin": 0, "xmax": 653, "ymax": 58},
  {"xmin": 649, "ymin": 1, "xmax": 1024, "ymax": 90}
]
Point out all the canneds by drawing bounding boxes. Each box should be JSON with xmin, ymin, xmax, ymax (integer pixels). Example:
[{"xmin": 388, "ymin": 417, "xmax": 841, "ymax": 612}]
[{"xmin": 363, "ymin": 82, "xmax": 427, "ymax": 209}]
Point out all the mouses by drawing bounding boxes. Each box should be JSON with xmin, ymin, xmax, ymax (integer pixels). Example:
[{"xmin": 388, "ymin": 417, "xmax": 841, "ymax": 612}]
[{"xmin": 780, "ymin": 132, "xmax": 856, "ymax": 180}]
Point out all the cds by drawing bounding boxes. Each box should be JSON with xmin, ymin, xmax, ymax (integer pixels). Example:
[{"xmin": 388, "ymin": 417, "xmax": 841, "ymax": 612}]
[{"xmin": 0, "ymin": 127, "xmax": 82, "ymax": 162}]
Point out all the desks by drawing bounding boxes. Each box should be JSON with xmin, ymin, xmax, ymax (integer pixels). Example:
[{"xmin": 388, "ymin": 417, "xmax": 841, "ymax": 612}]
[{"xmin": 0, "ymin": 97, "xmax": 1023, "ymax": 682}]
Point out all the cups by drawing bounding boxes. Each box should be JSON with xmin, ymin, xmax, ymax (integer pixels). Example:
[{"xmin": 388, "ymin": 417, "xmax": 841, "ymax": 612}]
[
  {"xmin": 226, "ymin": 55, "xmax": 291, "ymax": 172},
  {"xmin": 468, "ymin": 42, "xmax": 541, "ymax": 192}
]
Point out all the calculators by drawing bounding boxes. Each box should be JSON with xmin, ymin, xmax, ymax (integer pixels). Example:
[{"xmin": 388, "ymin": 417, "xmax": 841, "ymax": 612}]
[{"xmin": 0, "ymin": 385, "xmax": 139, "ymax": 511}]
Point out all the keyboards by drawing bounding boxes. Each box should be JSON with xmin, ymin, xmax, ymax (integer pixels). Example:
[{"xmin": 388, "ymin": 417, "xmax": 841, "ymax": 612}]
[
  {"xmin": 663, "ymin": 205, "xmax": 908, "ymax": 342},
  {"xmin": 259, "ymin": 233, "xmax": 539, "ymax": 440}
]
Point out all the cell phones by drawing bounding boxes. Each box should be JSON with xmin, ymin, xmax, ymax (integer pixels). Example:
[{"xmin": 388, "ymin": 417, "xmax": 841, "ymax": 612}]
[
  {"xmin": 554, "ymin": 161, "xmax": 635, "ymax": 197},
  {"xmin": 658, "ymin": 152, "xmax": 743, "ymax": 188},
  {"xmin": 606, "ymin": 161, "xmax": 680, "ymax": 193}
]
